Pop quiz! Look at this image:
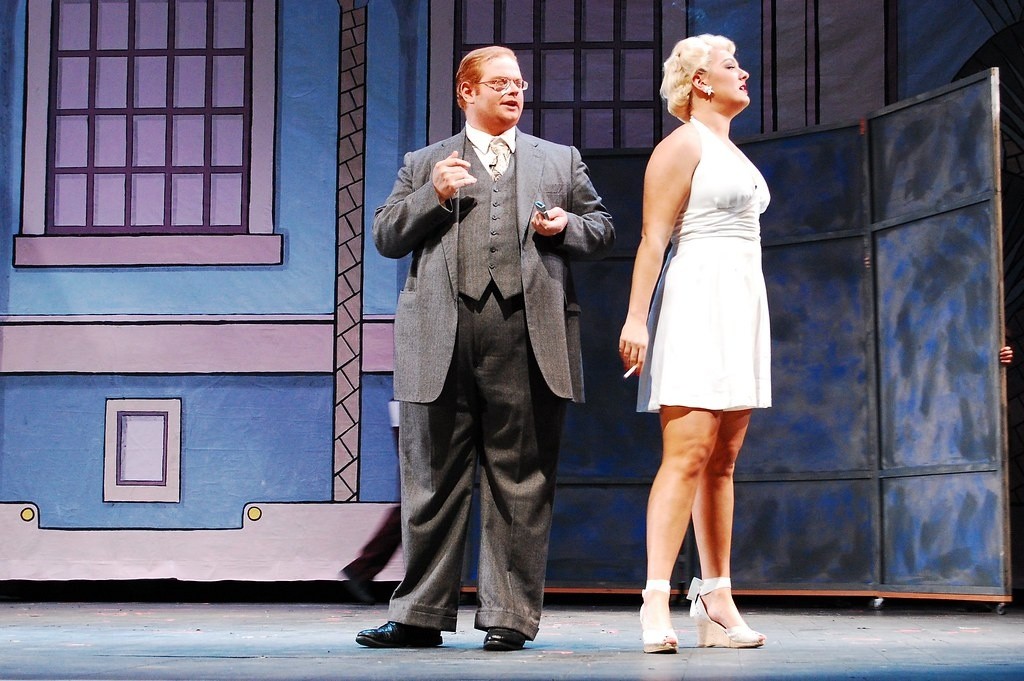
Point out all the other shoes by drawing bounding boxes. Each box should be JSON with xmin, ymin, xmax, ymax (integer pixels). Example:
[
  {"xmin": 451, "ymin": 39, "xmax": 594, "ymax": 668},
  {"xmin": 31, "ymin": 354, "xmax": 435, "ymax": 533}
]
[{"xmin": 341, "ymin": 565, "xmax": 375, "ymax": 605}]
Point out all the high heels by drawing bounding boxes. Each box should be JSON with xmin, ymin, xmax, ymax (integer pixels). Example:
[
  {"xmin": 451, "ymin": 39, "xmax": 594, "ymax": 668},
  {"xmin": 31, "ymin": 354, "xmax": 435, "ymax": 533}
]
[
  {"xmin": 686, "ymin": 577, "xmax": 763, "ymax": 648},
  {"xmin": 639, "ymin": 581, "xmax": 679, "ymax": 653}
]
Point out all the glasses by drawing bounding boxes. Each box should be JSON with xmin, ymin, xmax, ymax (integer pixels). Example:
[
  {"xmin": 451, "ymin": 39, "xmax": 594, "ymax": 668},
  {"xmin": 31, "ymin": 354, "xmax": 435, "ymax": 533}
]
[{"xmin": 471, "ymin": 78, "xmax": 529, "ymax": 92}]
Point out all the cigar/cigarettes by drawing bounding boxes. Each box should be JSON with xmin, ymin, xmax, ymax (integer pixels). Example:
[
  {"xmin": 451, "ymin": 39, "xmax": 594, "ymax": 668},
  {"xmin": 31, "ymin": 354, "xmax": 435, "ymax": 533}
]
[{"xmin": 623, "ymin": 364, "xmax": 637, "ymax": 379}]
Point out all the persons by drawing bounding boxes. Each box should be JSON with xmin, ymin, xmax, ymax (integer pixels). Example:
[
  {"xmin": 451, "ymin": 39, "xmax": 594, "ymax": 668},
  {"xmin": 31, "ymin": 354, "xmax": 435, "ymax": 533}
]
[
  {"xmin": 355, "ymin": 47, "xmax": 616, "ymax": 651},
  {"xmin": 620, "ymin": 35, "xmax": 772, "ymax": 653}
]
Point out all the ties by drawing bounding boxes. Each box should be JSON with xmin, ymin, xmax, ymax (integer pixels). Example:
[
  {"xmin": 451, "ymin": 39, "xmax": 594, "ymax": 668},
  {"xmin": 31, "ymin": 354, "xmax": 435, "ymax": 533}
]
[{"xmin": 491, "ymin": 144, "xmax": 509, "ymax": 180}]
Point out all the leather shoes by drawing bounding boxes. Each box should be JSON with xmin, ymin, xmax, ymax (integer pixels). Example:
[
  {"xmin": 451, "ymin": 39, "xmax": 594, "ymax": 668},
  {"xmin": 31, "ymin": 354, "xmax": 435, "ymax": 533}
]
[
  {"xmin": 484, "ymin": 627, "xmax": 526, "ymax": 650},
  {"xmin": 356, "ymin": 623, "xmax": 443, "ymax": 647}
]
